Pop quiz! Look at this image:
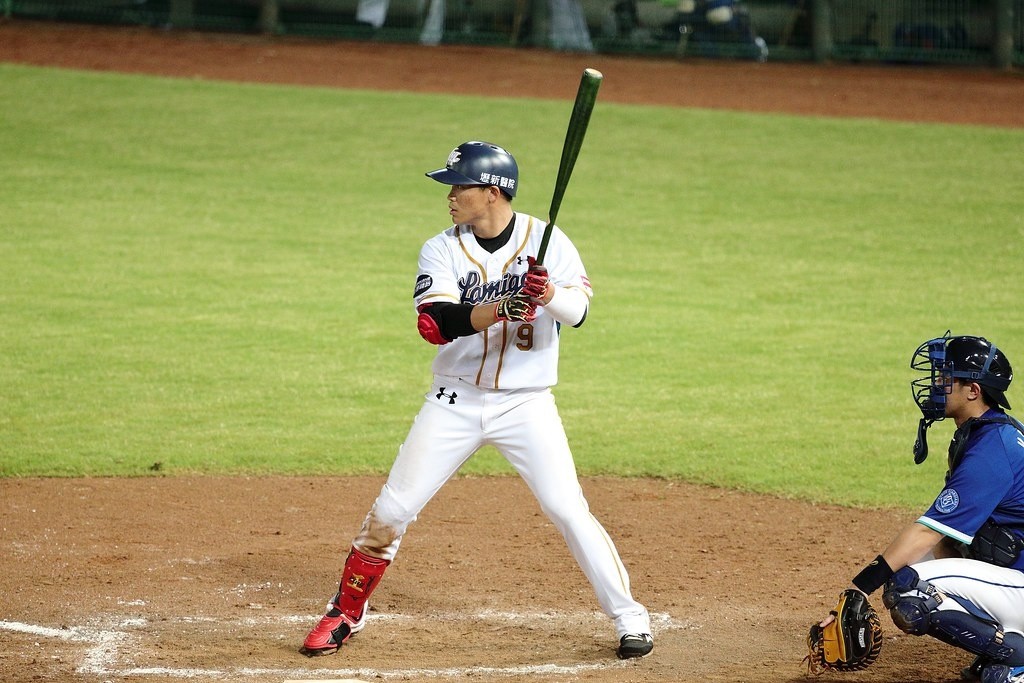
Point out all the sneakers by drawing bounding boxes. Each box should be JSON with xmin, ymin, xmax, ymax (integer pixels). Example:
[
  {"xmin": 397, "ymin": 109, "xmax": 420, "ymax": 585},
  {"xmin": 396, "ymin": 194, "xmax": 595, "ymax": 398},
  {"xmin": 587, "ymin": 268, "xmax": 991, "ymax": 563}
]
[
  {"xmin": 309, "ymin": 608, "xmax": 364, "ymax": 656},
  {"xmin": 617, "ymin": 633, "xmax": 654, "ymax": 658}
]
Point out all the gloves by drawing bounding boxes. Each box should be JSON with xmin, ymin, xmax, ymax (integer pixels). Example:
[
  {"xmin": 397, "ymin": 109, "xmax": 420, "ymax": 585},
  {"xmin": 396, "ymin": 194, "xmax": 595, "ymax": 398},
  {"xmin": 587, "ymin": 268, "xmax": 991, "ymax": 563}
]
[
  {"xmin": 494, "ymin": 297, "xmax": 538, "ymax": 323},
  {"xmin": 522, "ymin": 255, "xmax": 550, "ymax": 299}
]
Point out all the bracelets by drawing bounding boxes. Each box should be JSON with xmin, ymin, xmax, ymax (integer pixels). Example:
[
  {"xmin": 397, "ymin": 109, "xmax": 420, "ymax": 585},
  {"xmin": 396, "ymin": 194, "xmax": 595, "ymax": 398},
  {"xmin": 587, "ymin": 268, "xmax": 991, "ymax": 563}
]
[{"xmin": 852, "ymin": 555, "xmax": 894, "ymax": 596}]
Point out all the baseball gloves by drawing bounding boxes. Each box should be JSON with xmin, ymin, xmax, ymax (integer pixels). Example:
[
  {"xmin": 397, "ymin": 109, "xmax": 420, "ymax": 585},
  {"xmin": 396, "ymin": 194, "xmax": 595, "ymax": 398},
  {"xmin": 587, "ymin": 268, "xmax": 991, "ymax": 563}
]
[{"xmin": 799, "ymin": 587, "xmax": 883, "ymax": 678}]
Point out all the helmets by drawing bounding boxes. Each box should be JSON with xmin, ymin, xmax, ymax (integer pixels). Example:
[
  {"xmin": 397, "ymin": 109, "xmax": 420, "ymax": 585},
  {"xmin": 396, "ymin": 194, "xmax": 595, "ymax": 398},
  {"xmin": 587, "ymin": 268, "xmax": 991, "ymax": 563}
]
[
  {"xmin": 944, "ymin": 335, "xmax": 1013, "ymax": 410},
  {"xmin": 425, "ymin": 141, "xmax": 518, "ymax": 197}
]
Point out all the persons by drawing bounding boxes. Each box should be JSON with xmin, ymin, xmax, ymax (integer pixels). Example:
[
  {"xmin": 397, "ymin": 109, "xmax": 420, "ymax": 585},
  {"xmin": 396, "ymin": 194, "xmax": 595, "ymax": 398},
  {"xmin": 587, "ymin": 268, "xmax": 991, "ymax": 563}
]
[
  {"xmin": 806, "ymin": 335, "xmax": 1024, "ymax": 683},
  {"xmin": 300, "ymin": 138, "xmax": 652, "ymax": 660}
]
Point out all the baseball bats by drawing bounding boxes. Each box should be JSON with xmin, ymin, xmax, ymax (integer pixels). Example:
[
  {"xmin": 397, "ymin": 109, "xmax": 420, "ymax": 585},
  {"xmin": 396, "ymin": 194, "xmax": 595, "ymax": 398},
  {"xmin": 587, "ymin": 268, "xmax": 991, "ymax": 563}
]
[{"xmin": 536, "ymin": 68, "xmax": 603, "ymax": 264}]
